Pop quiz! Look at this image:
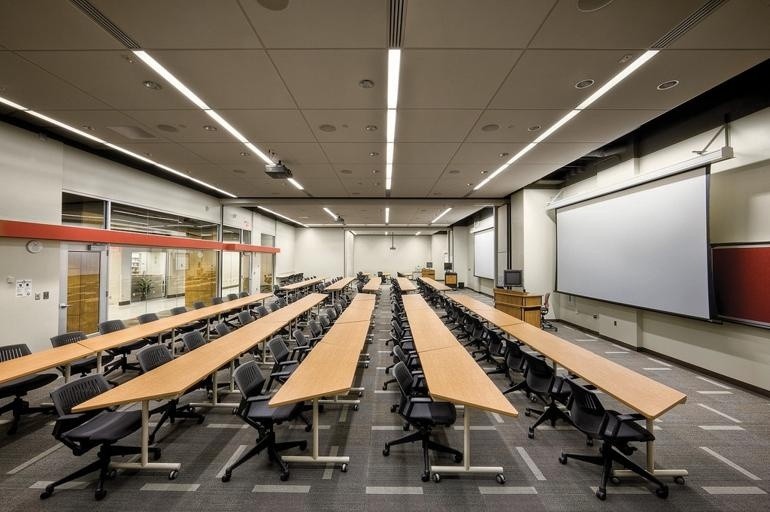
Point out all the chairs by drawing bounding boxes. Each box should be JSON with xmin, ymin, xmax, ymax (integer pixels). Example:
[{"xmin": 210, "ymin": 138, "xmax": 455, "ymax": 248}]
[
  {"xmin": 383, "ymin": 270, "xmax": 690, "ymax": 502},
  {"xmin": 1, "ymin": 272, "xmax": 382, "ymax": 501}
]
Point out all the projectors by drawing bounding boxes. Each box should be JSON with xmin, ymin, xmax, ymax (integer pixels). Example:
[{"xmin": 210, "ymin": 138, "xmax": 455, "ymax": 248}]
[
  {"xmin": 265, "ymin": 166, "xmax": 293, "ymax": 179},
  {"xmin": 337, "ymin": 219, "xmax": 344, "ymax": 224},
  {"xmin": 390, "ymin": 248, "xmax": 396, "ymax": 249}
]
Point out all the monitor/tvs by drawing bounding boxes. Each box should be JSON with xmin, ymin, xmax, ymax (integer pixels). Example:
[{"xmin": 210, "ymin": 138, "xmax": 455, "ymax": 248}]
[
  {"xmin": 444, "ymin": 263, "xmax": 452, "ymax": 272},
  {"xmin": 504, "ymin": 270, "xmax": 522, "ymax": 292},
  {"xmin": 427, "ymin": 262, "xmax": 432, "ymax": 269}
]
